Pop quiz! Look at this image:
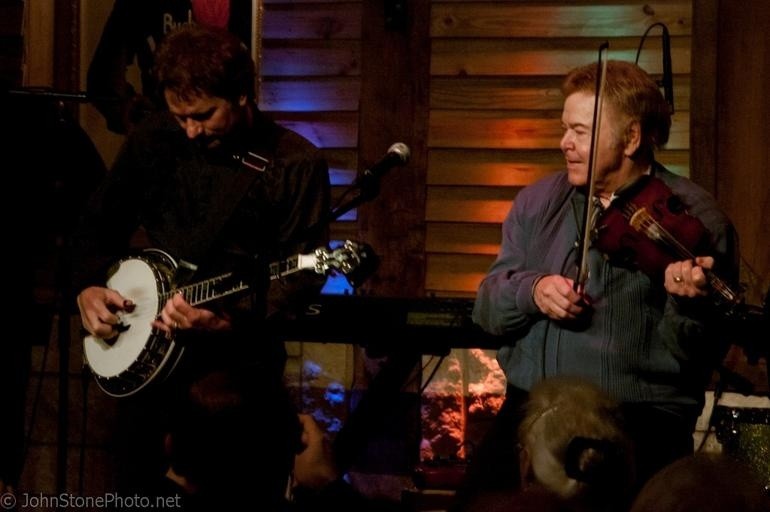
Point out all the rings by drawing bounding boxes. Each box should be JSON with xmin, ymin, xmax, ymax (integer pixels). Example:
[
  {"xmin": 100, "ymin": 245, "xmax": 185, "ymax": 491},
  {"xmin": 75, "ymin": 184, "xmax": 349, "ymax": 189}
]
[
  {"xmin": 673, "ymin": 276, "xmax": 682, "ymax": 283},
  {"xmin": 173, "ymin": 321, "xmax": 178, "ymax": 330}
]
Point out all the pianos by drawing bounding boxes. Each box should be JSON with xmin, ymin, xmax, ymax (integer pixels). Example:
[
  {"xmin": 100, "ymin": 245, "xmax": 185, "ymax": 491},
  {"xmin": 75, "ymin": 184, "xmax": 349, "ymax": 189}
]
[{"xmin": 288, "ymin": 289, "xmax": 500, "ymax": 351}]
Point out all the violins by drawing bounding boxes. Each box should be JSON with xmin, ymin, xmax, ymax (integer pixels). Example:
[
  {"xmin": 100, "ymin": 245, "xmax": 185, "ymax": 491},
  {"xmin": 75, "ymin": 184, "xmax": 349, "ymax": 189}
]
[{"xmin": 589, "ymin": 173, "xmax": 747, "ymax": 310}]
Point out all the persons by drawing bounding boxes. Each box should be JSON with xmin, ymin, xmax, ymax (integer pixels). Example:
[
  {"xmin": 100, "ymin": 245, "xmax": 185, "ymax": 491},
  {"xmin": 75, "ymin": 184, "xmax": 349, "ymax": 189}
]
[
  {"xmin": 513, "ymin": 376, "xmax": 632, "ymax": 507},
  {"xmin": 628, "ymin": 453, "xmax": 769, "ymax": 511},
  {"xmin": 448, "ymin": 56, "xmax": 746, "ymax": 509},
  {"xmin": 84, "ymin": 0, "xmax": 199, "ymax": 136},
  {"xmin": 64, "ymin": 27, "xmax": 335, "ymax": 510}
]
